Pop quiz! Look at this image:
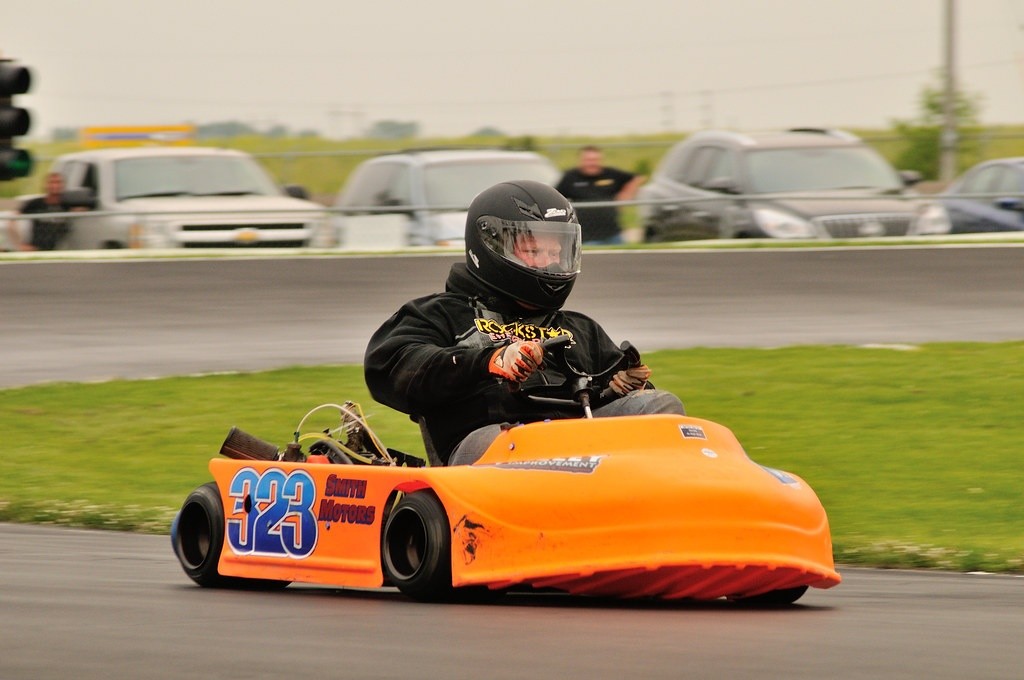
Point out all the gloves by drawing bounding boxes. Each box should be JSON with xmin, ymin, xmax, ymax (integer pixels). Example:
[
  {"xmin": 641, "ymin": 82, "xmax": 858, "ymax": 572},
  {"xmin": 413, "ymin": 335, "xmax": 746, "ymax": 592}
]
[
  {"xmin": 609, "ymin": 365, "xmax": 651, "ymax": 396},
  {"xmin": 487, "ymin": 340, "xmax": 544, "ymax": 384}
]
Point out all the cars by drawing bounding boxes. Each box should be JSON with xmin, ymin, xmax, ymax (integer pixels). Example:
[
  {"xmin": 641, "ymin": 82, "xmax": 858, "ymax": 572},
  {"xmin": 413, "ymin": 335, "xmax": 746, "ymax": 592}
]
[
  {"xmin": 311, "ymin": 149, "xmax": 566, "ymax": 248},
  {"xmin": 930, "ymin": 157, "xmax": 1022, "ymax": 234},
  {"xmin": 638, "ymin": 125, "xmax": 955, "ymax": 238}
]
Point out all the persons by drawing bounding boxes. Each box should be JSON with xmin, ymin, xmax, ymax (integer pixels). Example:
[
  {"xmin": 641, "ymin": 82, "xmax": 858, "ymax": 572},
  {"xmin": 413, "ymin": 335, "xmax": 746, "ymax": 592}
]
[
  {"xmin": 364, "ymin": 182, "xmax": 684, "ymax": 467},
  {"xmin": 555, "ymin": 145, "xmax": 647, "ymax": 249},
  {"xmin": 7, "ymin": 172, "xmax": 97, "ymax": 252}
]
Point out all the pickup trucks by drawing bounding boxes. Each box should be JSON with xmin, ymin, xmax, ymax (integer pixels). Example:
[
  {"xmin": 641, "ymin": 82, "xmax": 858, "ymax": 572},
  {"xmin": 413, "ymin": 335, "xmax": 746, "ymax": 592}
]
[{"xmin": 0, "ymin": 145, "xmax": 326, "ymax": 255}]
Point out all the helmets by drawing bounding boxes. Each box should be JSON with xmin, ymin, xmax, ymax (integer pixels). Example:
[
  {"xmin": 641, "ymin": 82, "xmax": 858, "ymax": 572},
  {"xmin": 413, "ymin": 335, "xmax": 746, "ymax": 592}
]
[{"xmin": 464, "ymin": 180, "xmax": 581, "ymax": 309}]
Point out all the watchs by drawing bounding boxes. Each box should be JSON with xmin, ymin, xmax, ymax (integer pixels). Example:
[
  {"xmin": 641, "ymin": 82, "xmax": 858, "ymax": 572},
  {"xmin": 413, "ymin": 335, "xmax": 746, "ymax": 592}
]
[{"xmin": 493, "ymin": 346, "xmax": 508, "ymax": 368}]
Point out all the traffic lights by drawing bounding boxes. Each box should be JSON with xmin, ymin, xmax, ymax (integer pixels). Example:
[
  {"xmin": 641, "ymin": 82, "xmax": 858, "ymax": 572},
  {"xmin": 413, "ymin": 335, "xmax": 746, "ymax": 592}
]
[{"xmin": 0, "ymin": 58, "xmax": 33, "ymax": 181}]
